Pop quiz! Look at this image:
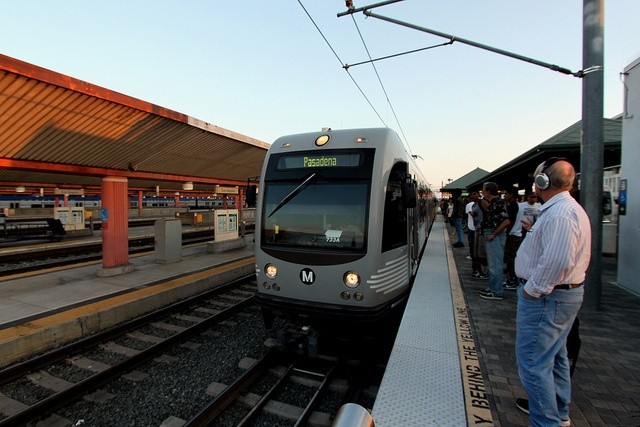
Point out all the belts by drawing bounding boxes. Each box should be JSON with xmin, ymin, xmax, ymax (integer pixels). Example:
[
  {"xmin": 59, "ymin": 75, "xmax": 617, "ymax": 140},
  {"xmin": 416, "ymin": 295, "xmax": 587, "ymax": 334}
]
[{"xmin": 519, "ymin": 278, "xmax": 583, "ymax": 288}]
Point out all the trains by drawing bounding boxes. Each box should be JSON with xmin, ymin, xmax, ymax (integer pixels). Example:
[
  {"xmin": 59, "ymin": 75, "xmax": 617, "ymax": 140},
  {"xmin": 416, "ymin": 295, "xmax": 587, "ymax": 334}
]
[{"xmin": 253, "ymin": 126, "xmax": 439, "ymax": 329}]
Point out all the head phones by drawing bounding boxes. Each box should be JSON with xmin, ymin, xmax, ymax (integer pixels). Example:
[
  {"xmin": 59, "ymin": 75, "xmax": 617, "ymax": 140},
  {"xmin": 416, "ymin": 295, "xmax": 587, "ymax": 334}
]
[{"xmin": 535, "ymin": 157, "xmax": 567, "ymax": 190}]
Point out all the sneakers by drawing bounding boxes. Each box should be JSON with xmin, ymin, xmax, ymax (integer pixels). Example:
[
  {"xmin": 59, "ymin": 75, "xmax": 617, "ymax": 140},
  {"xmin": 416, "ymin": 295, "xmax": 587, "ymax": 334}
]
[
  {"xmin": 476, "ymin": 274, "xmax": 490, "ymax": 279},
  {"xmin": 480, "ymin": 294, "xmax": 503, "ymax": 300},
  {"xmin": 453, "ymin": 242, "xmax": 464, "ymax": 248},
  {"xmin": 473, "ymin": 272, "xmax": 480, "ymax": 277},
  {"xmin": 516, "ymin": 398, "xmax": 571, "ymax": 426},
  {"xmin": 466, "ymin": 256, "xmax": 472, "ymax": 260},
  {"xmin": 481, "ymin": 288, "xmax": 490, "ymax": 293},
  {"xmin": 503, "ymin": 284, "xmax": 516, "ymax": 289},
  {"xmin": 503, "ymin": 281, "xmax": 511, "ymax": 284}
]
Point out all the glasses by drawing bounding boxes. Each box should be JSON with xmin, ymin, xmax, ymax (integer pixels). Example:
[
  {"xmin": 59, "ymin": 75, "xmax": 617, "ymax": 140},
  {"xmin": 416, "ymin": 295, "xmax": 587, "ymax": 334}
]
[{"xmin": 504, "ymin": 192, "xmax": 507, "ymax": 195}]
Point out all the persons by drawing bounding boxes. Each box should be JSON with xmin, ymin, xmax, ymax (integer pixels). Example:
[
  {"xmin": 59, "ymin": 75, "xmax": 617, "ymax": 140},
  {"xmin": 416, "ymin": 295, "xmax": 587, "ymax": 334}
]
[
  {"xmin": 508, "ymin": 157, "xmax": 593, "ymax": 427},
  {"xmin": 515, "ymin": 316, "xmax": 581, "ymax": 415},
  {"xmin": 440, "ymin": 181, "xmax": 543, "ymax": 302},
  {"xmin": 569, "ymin": 177, "xmax": 580, "ymax": 204}
]
